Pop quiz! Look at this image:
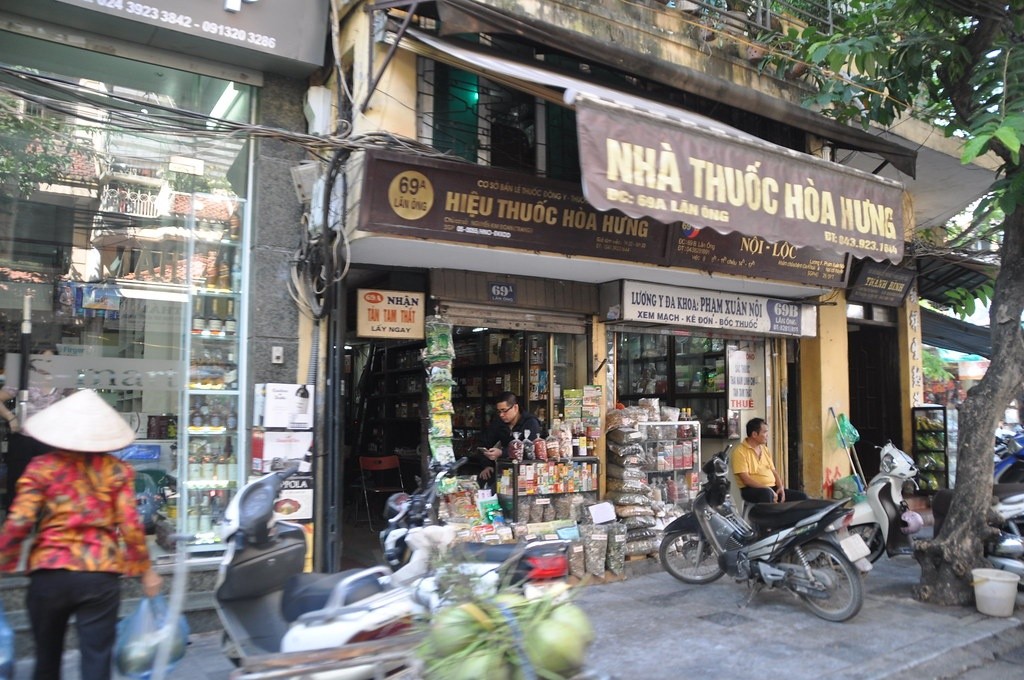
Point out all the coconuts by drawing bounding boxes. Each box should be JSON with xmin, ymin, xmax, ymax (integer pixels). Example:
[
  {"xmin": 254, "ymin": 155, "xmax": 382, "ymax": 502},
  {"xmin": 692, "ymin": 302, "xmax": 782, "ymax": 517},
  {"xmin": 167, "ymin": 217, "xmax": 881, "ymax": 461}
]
[{"xmin": 433, "ymin": 591, "xmax": 592, "ymax": 680}]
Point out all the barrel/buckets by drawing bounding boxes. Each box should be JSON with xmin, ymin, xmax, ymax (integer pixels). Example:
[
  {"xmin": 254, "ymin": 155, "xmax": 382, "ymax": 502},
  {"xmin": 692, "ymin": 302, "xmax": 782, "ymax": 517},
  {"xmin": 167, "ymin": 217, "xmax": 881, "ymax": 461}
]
[{"xmin": 969, "ymin": 568, "xmax": 1020, "ymax": 617}]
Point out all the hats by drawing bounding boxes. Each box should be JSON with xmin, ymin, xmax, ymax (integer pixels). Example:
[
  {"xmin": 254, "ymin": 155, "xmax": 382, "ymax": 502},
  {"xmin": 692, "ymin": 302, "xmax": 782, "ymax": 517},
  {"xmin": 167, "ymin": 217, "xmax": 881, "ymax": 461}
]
[{"xmin": 23, "ymin": 389, "xmax": 136, "ymax": 452}]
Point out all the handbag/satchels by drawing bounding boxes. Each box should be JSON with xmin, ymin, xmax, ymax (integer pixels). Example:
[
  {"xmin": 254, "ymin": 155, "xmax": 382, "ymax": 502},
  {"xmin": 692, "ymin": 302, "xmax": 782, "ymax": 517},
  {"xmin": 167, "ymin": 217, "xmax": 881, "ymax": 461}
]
[
  {"xmin": 111, "ymin": 595, "xmax": 189, "ymax": 680},
  {"xmin": 0, "ymin": 605, "xmax": 16, "ymax": 680}
]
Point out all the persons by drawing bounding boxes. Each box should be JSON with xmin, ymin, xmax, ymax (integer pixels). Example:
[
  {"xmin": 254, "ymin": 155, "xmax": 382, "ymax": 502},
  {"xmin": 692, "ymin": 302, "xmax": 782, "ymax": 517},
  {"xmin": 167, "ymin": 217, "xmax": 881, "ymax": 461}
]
[
  {"xmin": 478, "ymin": 391, "xmax": 541, "ymax": 495},
  {"xmin": 0, "ymin": 388, "xmax": 164, "ymax": 679},
  {"xmin": 0, "ymin": 341, "xmax": 78, "ymax": 518},
  {"xmin": 733, "ymin": 417, "xmax": 811, "ymax": 503}
]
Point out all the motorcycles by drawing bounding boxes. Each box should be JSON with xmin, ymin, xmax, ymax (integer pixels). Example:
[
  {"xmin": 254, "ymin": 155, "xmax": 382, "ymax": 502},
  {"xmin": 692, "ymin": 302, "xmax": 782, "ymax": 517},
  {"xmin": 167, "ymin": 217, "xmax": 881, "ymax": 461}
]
[
  {"xmin": 993, "ymin": 421, "xmax": 1024, "ymax": 486},
  {"xmin": 660, "ymin": 445, "xmax": 874, "ymax": 624}
]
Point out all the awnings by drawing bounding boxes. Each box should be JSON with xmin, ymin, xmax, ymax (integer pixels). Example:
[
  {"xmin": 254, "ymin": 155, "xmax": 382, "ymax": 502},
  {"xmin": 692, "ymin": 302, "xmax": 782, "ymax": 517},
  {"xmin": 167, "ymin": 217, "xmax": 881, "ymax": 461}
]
[
  {"xmin": 915, "ymin": 250, "xmax": 1003, "ymax": 360},
  {"xmin": 376, "ymin": 0, "xmax": 918, "ymax": 264}
]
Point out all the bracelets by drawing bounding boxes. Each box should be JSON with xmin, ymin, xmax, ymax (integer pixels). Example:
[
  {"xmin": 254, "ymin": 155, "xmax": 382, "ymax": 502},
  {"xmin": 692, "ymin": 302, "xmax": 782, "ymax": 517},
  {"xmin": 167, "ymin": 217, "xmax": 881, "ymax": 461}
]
[{"xmin": 8, "ymin": 416, "xmax": 16, "ymax": 422}]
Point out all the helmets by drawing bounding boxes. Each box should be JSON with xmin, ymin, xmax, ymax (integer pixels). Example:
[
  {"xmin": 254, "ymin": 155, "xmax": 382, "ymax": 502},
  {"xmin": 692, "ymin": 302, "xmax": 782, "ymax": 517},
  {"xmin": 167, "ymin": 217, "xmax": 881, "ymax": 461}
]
[{"xmin": 901, "ymin": 511, "xmax": 923, "ymax": 534}]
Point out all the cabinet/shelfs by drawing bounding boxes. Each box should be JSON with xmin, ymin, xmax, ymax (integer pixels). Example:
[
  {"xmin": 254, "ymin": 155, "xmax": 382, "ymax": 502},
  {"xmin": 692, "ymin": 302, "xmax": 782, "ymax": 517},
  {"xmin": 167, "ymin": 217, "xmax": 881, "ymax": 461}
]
[
  {"xmin": 911, "ymin": 405, "xmax": 948, "ymax": 492},
  {"xmin": 616, "ymin": 333, "xmax": 725, "ymax": 511},
  {"xmin": 152, "ymin": 233, "xmax": 247, "ymax": 550},
  {"xmin": 365, "ymin": 330, "xmax": 601, "ymax": 526}
]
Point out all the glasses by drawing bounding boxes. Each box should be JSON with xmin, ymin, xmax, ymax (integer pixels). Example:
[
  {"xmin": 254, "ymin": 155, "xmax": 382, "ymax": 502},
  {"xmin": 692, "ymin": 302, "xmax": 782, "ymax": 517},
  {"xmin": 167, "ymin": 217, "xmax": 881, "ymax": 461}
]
[{"xmin": 496, "ymin": 402, "xmax": 518, "ymax": 414}]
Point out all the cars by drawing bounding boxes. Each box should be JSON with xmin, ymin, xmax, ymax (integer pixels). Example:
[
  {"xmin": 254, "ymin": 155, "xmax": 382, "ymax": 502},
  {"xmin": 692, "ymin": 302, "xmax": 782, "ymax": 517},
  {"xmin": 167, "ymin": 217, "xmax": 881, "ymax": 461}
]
[{"xmin": 134, "ymin": 473, "xmax": 164, "ymax": 532}]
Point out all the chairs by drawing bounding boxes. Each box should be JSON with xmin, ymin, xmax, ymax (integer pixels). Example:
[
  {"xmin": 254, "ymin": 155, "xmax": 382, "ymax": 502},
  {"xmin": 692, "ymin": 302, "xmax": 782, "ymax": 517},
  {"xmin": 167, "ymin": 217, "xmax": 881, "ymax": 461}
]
[{"xmin": 351, "ymin": 455, "xmax": 407, "ymax": 532}]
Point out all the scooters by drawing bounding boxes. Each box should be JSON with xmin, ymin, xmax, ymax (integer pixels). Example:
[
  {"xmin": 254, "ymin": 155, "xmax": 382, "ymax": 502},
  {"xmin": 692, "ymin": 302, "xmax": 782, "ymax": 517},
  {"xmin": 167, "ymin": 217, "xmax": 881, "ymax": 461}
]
[
  {"xmin": 843, "ymin": 438, "xmax": 1024, "ymax": 587},
  {"xmin": 209, "ymin": 464, "xmax": 587, "ymax": 680}
]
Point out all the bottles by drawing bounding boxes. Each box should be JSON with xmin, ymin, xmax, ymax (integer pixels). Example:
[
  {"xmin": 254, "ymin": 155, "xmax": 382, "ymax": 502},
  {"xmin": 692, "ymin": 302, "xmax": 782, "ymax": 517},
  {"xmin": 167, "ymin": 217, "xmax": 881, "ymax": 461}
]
[
  {"xmin": 175, "ymin": 247, "xmax": 238, "ymax": 533},
  {"xmin": 571, "ymin": 421, "xmax": 595, "ymax": 456},
  {"xmin": 679, "ymin": 408, "xmax": 692, "ymax": 421}
]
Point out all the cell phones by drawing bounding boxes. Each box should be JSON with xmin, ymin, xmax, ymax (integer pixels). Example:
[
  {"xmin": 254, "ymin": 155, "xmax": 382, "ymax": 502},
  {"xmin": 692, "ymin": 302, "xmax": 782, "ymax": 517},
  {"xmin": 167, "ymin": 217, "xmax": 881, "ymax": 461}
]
[{"xmin": 477, "ymin": 447, "xmax": 489, "ymax": 453}]
